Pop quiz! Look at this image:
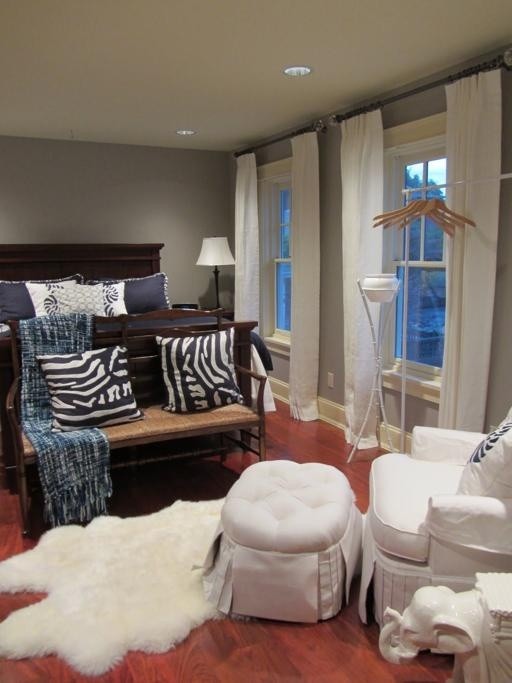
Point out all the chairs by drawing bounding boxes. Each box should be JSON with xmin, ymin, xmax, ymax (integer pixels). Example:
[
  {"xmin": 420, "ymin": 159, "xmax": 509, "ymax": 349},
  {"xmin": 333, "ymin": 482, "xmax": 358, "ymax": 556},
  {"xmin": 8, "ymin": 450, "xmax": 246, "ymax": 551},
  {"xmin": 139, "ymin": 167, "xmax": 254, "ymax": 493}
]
[{"xmin": 357, "ymin": 424, "xmax": 512, "ymax": 630}]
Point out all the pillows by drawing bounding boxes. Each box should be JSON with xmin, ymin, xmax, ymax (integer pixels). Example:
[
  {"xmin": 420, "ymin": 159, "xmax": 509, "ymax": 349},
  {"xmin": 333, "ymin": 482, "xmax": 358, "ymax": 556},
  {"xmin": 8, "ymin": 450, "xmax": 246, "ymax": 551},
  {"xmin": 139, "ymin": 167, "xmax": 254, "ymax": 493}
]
[
  {"xmin": 1, "ymin": 271, "xmax": 172, "ymax": 324},
  {"xmin": 36, "ymin": 325, "xmax": 246, "ymax": 433},
  {"xmin": 454, "ymin": 402, "xmax": 512, "ymax": 497}
]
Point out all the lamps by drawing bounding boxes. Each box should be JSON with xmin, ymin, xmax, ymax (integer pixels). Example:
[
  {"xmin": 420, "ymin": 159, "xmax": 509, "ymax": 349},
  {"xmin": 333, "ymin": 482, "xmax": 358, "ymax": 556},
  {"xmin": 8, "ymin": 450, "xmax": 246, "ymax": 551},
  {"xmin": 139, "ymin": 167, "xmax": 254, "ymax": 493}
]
[{"xmin": 195, "ymin": 233, "xmax": 238, "ymax": 311}]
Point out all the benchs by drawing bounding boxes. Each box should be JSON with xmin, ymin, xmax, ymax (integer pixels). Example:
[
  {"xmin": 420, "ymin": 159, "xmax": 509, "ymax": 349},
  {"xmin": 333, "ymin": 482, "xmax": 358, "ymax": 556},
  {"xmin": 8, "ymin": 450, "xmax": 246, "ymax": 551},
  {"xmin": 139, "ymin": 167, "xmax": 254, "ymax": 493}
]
[{"xmin": 4, "ymin": 316, "xmax": 268, "ymax": 538}]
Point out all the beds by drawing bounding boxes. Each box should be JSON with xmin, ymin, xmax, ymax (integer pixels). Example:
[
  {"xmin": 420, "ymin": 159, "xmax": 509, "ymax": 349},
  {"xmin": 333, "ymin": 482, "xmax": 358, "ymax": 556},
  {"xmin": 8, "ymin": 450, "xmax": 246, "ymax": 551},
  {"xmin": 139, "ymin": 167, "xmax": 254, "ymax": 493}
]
[{"xmin": 1, "ymin": 241, "xmax": 260, "ymax": 494}]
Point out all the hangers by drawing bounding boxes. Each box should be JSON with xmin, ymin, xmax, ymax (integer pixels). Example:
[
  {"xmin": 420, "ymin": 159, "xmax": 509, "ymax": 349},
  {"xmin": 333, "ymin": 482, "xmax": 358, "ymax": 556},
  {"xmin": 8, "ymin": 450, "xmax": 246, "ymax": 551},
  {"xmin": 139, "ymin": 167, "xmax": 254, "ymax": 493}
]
[{"xmin": 371, "ymin": 183, "xmax": 476, "ymax": 236}]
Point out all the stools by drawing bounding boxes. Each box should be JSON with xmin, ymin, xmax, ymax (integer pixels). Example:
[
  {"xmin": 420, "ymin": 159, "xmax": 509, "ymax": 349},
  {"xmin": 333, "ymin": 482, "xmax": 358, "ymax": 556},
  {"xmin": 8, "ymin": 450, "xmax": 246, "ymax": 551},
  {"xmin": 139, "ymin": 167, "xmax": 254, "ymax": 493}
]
[{"xmin": 190, "ymin": 458, "xmax": 364, "ymax": 625}]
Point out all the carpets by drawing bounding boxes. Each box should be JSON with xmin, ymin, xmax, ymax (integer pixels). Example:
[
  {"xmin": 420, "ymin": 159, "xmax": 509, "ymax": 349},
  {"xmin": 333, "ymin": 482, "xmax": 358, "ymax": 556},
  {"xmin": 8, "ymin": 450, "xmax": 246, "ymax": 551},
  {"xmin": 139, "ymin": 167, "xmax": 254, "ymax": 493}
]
[{"xmin": 1, "ymin": 496, "xmax": 236, "ymax": 678}]
